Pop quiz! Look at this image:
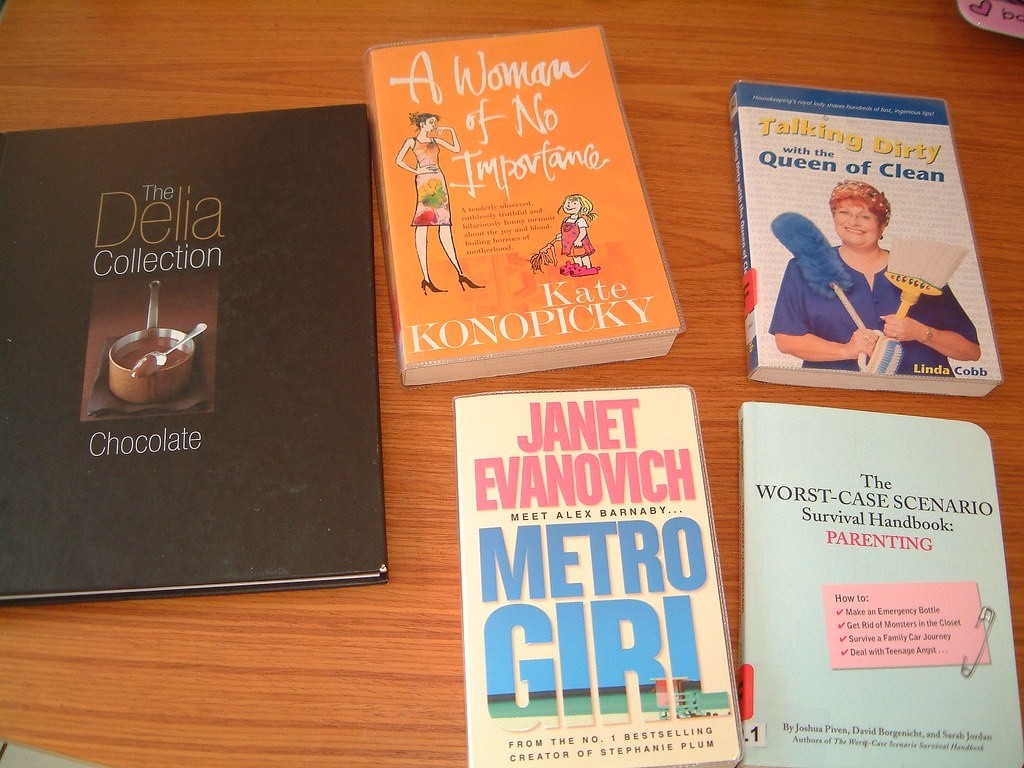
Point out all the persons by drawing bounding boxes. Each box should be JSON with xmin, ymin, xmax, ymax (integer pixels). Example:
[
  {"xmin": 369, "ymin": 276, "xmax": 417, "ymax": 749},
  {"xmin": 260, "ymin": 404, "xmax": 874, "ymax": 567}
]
[{"xmin": 769, "ymin": 179, "xmax": 981, "ymax": 376}]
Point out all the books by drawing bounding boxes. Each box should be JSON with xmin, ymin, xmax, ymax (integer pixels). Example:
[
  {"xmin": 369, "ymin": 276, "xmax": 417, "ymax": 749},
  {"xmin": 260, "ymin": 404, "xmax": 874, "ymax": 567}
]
[
  {"xmin": 0, "ymin": 104, "xmax": 390, "ymax": 601},
  {"xmin": 729, "ymin": 80, "xmax": 1003, "ymax": 397},
  {"xmin": 738, "ymin": 402, "xmax": 1023, "ymax": 768},
  {"xmin": 451, "ymin": 385, "xmax": 742, "ymax": 768},
  {"xmin": 363, "ymin": 24, "xmax": 686, "ymax": 388}
]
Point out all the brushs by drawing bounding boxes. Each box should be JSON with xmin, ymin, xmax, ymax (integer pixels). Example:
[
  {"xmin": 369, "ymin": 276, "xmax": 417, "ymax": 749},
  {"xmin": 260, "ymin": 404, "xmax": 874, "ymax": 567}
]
[{"xmin": 857, "ymin": 328, "xmax": 904, "ymax": 376}]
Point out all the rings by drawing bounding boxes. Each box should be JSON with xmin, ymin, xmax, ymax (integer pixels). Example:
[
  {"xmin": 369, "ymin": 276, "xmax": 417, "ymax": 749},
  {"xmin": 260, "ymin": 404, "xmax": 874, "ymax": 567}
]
[
  {"xmin": 866, "ymin": 335, "xmax": 870, "ymax": 340},
  {"xmin": 893, "ymin": 333, "xmax": 895, "ymax": 336}
]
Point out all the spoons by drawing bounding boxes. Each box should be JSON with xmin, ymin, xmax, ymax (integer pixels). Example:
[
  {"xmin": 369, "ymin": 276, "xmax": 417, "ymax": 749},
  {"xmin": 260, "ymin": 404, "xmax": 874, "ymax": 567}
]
[{"xmin": 129, "ymin": 323, "xmax": 209, "ymax": 378}]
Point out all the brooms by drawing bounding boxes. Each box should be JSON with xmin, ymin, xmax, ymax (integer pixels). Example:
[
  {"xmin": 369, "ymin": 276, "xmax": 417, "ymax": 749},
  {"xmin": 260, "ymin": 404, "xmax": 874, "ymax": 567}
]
[{"xmin": 883, "ymin": 235, "xmax": 969, "ymax": 318}]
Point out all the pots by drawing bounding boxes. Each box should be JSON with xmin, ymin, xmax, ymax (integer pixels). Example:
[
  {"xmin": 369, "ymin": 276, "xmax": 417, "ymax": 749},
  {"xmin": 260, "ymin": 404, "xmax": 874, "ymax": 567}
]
[{"xmin": 108, "ymin": 280, "xmax": 195, "ymax": 403}]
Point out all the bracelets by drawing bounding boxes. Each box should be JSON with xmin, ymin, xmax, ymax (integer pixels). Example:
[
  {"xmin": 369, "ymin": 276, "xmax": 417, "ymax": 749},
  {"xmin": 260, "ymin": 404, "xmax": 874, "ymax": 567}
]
[{"xmin": 923, "ymin": 327, "xmax": 932, "ymax": 343}]
